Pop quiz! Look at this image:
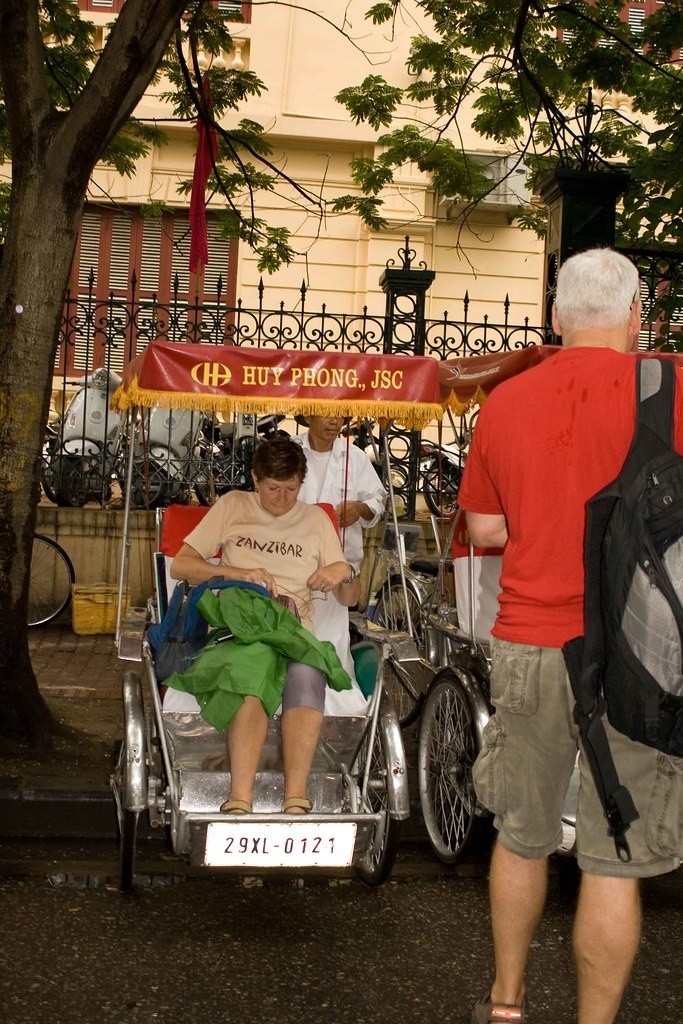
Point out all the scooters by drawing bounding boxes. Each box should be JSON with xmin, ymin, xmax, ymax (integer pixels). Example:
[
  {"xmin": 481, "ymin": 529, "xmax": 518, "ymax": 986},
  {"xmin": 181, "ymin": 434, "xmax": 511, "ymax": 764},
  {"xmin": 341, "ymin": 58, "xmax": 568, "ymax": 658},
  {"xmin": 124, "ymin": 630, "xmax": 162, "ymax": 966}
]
[
  {"xmin": 40, "ymin": 365, "xmax": 380, "ymax": 507},
  {"xmin": 413, "ymin": 421, "xmax": 480, "ymax": 519}
]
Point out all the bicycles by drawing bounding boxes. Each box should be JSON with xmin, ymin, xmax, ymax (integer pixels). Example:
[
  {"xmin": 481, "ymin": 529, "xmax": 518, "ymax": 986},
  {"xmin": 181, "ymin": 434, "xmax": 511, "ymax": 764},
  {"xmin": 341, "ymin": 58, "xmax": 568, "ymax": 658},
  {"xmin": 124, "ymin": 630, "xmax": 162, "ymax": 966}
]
[{"xmin": 25, "ymin": 531, "xmax": 78, "ymax": 628}]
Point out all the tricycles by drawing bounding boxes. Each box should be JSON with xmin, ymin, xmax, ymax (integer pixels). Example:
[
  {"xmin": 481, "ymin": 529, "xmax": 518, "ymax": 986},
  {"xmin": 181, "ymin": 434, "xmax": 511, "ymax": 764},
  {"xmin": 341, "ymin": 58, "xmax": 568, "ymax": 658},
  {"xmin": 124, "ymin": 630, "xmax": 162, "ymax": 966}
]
[
  {"xmin": 369, "ymin": 513, "xmax": 502, "ymax": 866},
  {"xmin": 105, "ymin": 496, "xmax": 408, "ymax": 894}
]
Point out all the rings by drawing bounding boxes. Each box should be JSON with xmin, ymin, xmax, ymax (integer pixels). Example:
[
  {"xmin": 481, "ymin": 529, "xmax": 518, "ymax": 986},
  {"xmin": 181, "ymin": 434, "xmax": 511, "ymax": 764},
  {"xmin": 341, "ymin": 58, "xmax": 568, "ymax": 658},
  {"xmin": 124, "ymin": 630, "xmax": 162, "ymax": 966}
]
[{"xmin": 321, "ymin": 584, "xmax": 325, "ymax": 588}]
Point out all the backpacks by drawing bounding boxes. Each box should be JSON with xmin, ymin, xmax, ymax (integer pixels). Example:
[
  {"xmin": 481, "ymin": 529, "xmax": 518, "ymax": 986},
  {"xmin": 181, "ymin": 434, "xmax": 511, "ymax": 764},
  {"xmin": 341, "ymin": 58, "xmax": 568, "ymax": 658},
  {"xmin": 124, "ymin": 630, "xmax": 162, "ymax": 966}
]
[{"xmin": 561, "ymin": 357, "xmax": 683, "ymax": 758}]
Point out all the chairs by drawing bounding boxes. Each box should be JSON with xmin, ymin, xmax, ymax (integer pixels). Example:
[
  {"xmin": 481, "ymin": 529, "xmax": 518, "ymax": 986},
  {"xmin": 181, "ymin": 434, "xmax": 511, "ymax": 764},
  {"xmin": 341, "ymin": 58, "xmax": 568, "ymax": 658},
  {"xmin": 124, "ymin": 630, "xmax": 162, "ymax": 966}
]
[{"xmin": 155, "ymin": 502, "xmax": 366, "ymax": 728}]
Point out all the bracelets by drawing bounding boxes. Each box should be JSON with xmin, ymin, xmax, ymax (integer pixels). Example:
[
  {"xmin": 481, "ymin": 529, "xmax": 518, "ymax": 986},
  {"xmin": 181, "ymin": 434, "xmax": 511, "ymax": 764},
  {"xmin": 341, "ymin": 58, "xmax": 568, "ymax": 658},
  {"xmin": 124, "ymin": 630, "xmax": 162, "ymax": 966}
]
[{"xmin": 343, "ymin": 567, "xmax": 356, "ymax": 583}]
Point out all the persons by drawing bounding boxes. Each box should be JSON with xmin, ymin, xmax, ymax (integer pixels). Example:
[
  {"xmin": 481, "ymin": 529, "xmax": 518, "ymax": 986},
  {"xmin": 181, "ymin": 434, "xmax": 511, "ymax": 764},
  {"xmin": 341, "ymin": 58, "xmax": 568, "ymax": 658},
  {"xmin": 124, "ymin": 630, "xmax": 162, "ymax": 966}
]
[
  {"xmin": 456, "ymin": 246, "xmax": 683, "ymax": 1024},
  {"xmin": 170, "ymin": 435, "xmax": 361, "ymax": 815},
  {"xmin": 290, "ymin": 416, "xmax": 387, "ymax": 643}
]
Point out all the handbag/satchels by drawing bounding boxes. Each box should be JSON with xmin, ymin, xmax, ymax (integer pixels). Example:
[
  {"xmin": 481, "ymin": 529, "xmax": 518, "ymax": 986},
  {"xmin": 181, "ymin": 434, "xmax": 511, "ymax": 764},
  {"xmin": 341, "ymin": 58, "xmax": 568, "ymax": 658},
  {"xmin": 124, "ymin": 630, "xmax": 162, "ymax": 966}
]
[{"xmin": 148, "ymin": 579, "xmax": 210, "ymax": 675}]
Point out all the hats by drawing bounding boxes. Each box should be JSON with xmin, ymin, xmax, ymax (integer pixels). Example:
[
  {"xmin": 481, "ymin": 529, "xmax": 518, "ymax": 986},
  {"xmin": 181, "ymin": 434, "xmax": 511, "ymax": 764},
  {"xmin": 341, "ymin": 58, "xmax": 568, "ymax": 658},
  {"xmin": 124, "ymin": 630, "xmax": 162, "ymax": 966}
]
[{"xmin": 293, "ymin": 415, "xmax": 353, "ymax": 427}]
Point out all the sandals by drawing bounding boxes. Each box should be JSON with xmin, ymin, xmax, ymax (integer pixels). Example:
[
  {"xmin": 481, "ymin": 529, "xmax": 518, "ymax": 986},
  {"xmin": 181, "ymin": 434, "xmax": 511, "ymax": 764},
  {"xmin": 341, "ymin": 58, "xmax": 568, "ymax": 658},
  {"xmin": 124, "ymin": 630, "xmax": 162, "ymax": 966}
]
[
  {"xmin": 280, "ymin": 795, "xmax": 313, "ymax": 814},
  {"xmin": 220, "ymin": 799, "xmax": 253, "ymax": 815},
  {"xmin": 470, "ymin": 988, "xmax": 528, "ymax": 1024}
]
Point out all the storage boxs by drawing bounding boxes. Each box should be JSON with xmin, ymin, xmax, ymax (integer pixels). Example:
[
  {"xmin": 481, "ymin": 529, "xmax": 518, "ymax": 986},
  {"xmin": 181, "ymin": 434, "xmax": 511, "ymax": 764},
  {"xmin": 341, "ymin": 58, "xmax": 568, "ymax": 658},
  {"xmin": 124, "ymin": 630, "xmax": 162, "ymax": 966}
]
[{"xmin": 70, "ymin": 580, "xmax": 132, "ymax": 635}]
